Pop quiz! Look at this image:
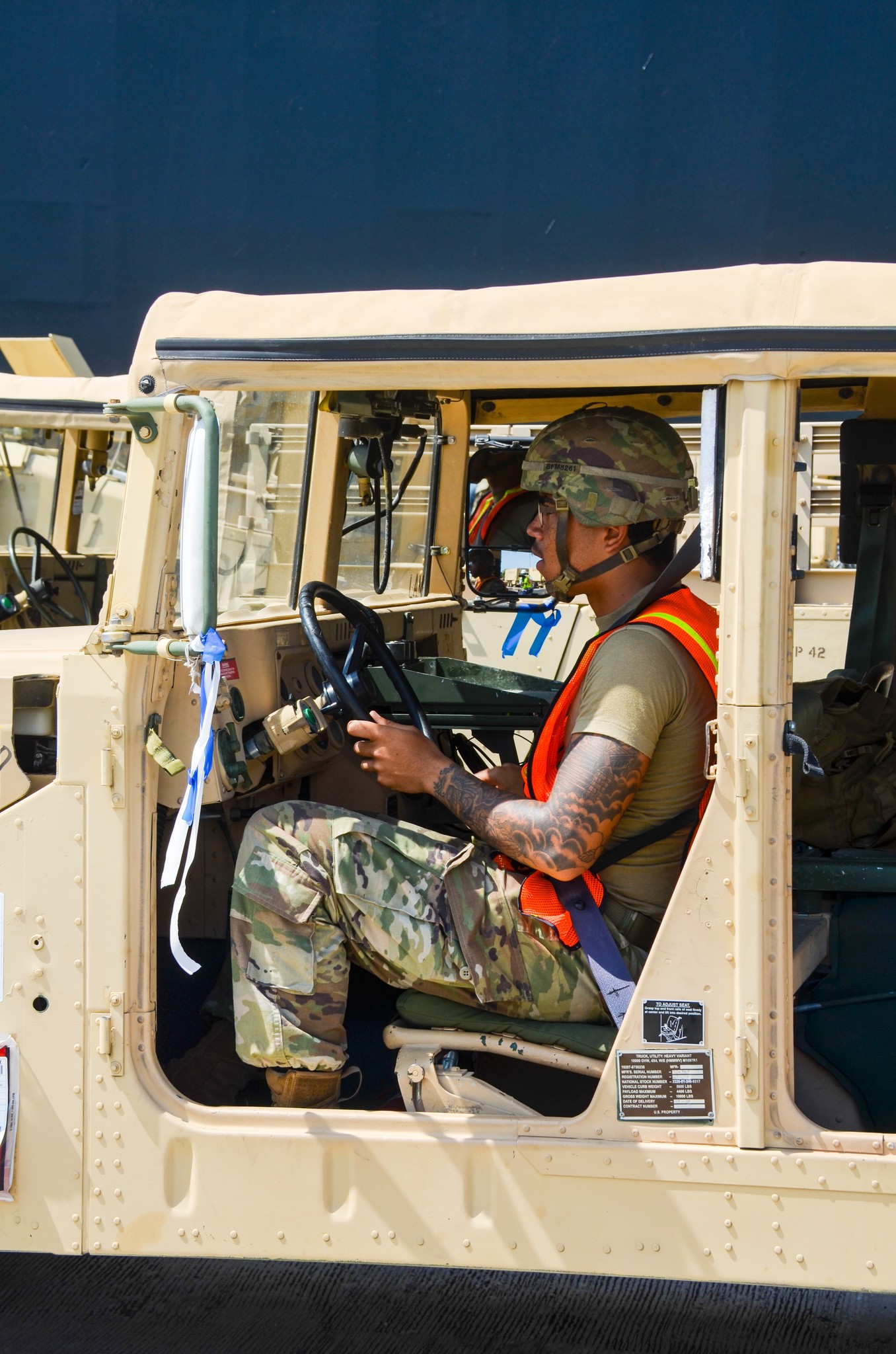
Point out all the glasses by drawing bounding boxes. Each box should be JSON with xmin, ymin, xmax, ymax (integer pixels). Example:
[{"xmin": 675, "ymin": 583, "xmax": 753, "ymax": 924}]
[{"xmin": 536, "ymin": 501, "xmax": 560, "ymax": 527}]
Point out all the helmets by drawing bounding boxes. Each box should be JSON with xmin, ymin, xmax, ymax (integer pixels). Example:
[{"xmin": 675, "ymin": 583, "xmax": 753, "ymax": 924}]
[{"xmin": 519, "ymin": 404, "xmax": 699, "ymax": 527}]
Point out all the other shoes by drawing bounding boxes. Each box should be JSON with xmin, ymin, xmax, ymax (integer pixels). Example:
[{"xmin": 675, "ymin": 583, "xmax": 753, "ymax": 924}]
[{"xmin": 261, "ymin": 1065, "xmax": 361, "ymax": 1109}]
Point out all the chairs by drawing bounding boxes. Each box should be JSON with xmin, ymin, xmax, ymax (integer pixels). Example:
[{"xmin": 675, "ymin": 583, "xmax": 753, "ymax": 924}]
[
  {"xmin": 382, "ymin": 991, "xmax": 605, "ymax": 1076},
  {"xmin": 789, "ymin": 416, "xmax": 896, "ymax": 890}
]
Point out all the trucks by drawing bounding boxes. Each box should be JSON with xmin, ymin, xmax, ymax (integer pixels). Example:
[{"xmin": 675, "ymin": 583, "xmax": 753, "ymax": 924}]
[{"xmin": 0, "ymin": 257, "xmax": 895, "ymax": 1304}]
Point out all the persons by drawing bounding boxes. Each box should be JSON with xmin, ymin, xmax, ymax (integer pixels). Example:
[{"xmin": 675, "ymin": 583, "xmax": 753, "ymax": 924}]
[
  {"xmin": 467, "ymin": 549, "xmax": 510, "ymax": 595},
  {"xmin": 518, "ymin": 569, "xmax": 533, "ymax": 590},
  {"xmin": 463, "ymin": 440, "xmax": 546, "ymax": 550},
  {"xmin": 228, "ymin": 401, "xmax": 717, "ymax": 1109}
]
